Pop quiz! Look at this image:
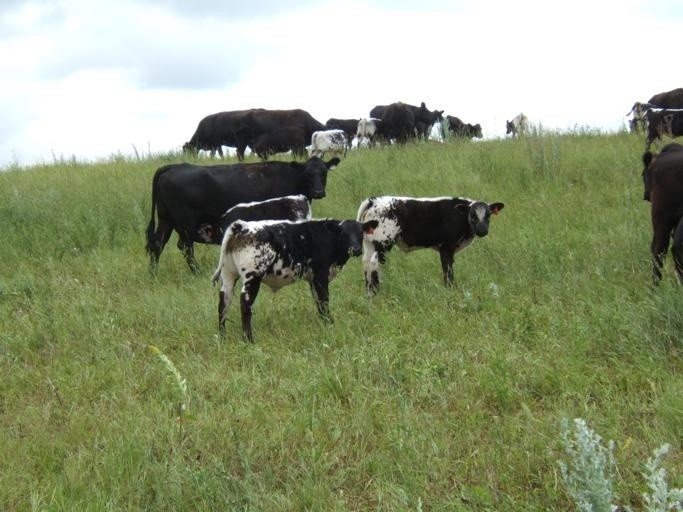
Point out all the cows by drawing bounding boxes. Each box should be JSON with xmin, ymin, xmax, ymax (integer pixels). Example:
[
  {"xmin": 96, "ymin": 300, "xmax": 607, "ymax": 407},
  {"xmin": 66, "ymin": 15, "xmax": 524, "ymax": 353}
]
[
  {"xmin": 642, "ymin": 143, "xmax": 683, "ymax": 294},
  {"xmin": 440, "ymin": 115, "xmax": 483, "ymax": 143},
  {"xmin": 145, "ymin": 156, "xmax": 340, "ymax": 293},
  {"xmin": 187, "ymin": 194, "xmax": 312, "ymax": 245},
  {"xmin": 183, "ymin": 102, "xmax": 444, "ymax": 164},
  {"xmin": 506, "ymin": 113, "xmax": 528, "ymax": 142},
  {"xmin": 212, "ymin": 219, "xmax": 379, "ymax": 344},
  {"xmin": 627, "ymin": 88, "xmax": 683, "ymax": 152},
  {"xmin": 356, "ymin": 196, "xmax": 504, "ymax": 301}
]
